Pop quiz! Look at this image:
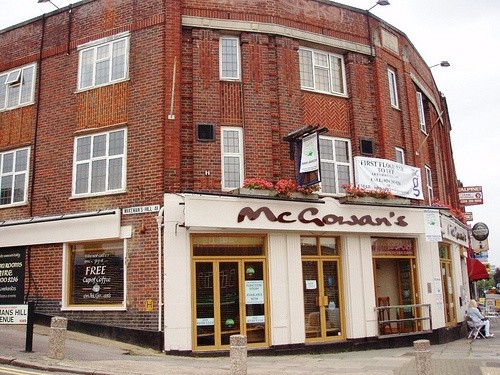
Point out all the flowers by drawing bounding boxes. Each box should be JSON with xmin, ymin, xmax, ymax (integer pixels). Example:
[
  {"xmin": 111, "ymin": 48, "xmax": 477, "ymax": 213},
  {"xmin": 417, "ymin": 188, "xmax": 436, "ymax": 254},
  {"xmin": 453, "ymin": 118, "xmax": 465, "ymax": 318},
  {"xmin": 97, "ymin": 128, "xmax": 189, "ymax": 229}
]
[
  {"xmin": 241, "ymin": 176, "xmax": 320, "ymax": 199},
  {"xmin": 433, "ymin": 198, "xmax": 468, "ymax": 225},
  {"xmin": 340, "ymin": 183, "xmax": 395, "ymax": 199}
]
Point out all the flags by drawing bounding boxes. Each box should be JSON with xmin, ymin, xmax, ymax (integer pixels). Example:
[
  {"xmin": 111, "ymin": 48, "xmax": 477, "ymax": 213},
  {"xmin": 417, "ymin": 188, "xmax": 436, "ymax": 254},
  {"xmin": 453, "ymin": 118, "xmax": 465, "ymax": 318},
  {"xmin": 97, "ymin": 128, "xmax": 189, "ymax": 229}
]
[{"xmin": 299, "ymin": 132, "xmax": 318, "ymax": 174}]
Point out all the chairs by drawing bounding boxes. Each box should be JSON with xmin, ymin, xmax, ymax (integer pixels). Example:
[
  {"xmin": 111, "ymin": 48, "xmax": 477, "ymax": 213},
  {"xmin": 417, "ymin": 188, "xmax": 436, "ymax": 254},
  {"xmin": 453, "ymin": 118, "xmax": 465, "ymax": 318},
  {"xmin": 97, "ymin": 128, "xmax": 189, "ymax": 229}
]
[{"xmin": 464, "ymin": 315, "xmax": 486, "ymax": 340}]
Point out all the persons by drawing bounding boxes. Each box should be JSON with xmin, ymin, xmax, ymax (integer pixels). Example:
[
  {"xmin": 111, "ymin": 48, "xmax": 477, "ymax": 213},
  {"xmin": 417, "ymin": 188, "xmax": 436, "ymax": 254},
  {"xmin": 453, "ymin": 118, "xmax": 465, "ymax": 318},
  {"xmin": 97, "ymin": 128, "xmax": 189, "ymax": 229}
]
[
  {"xmin": 467, "ymin": 299, "xmax": 493, "ymax": 338},
  {"xmin": 473, "ymin": 300, "xmax": 485, "ymax": 338}
]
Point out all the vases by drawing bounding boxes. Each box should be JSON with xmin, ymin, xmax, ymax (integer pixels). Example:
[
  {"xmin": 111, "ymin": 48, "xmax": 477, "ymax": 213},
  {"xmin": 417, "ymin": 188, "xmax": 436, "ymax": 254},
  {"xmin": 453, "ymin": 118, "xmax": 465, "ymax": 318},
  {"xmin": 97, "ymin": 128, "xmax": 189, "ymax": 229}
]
[
  {"xmin": 340, "ymin": 196, "xmax": 411, "ymax": 206},
  {"xmin": 227, "ymin": 188, "xmax": 319, "ymax": 201}
]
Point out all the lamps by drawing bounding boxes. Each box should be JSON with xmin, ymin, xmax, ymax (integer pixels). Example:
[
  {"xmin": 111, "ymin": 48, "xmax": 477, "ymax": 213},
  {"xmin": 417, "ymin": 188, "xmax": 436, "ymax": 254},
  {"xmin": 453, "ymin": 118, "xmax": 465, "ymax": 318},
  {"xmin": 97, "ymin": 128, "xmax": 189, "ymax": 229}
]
[
  {"xmin": 367, "ymin": 0, "xmax": 390, "ymax": 13},
  {"xmin": 37, "ymin": 0, "xmax": 59, "ymax": 11},
  {"xmin": 429, "ymin": 60, "xmax": 451, "ymax": 70}
]
